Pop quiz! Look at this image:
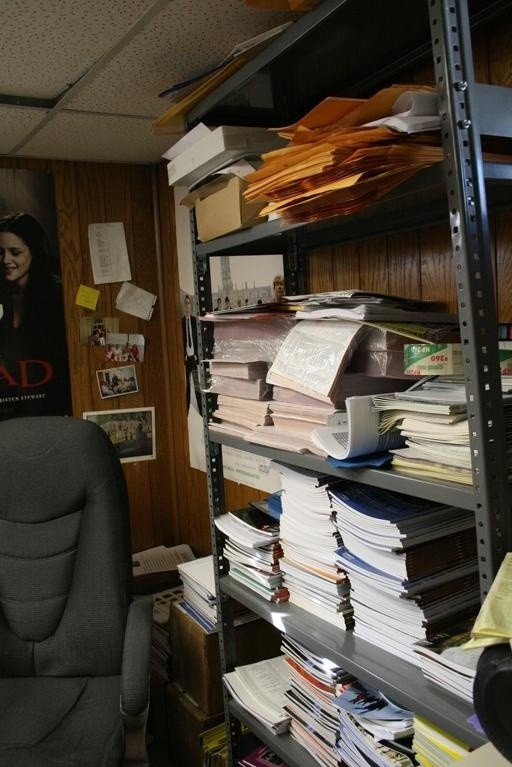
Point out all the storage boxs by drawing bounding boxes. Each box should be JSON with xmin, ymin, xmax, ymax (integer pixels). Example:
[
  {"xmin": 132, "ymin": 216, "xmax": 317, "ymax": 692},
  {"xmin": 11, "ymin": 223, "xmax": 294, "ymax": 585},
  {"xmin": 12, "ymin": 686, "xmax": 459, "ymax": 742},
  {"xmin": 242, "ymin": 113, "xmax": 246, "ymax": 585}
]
[{"xmin": 146, "ymin": 594, "xmax": 282, "ymax": 767}]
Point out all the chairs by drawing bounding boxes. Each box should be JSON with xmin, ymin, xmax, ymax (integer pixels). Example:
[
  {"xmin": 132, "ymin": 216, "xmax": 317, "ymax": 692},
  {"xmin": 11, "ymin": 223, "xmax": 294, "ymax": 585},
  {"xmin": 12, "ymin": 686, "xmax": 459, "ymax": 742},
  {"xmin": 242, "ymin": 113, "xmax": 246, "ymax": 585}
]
[{"xmin": 1, "ymin": 418, "xmax": 156, "ymax": 765}]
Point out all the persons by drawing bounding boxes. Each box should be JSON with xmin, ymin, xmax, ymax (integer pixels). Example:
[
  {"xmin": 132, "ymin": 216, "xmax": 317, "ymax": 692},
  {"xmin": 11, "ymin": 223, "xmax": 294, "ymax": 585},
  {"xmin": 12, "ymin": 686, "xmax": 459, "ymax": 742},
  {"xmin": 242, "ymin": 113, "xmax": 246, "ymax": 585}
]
[
  {"xmin": 0, "ymin": 213, "xmax": 72, "ymax": 422},
  {"xmin": 105, "ymin": 341, "xmax": 139, "ymax": 361},
  {"xmin": 214, "ymin": 276, "xmax": 285, "ymax": 311},
  {"xmin": 136, "ymin": 423, "xmax": 147, "ymax": 456},
  {"xmin": 88, "ymin": 327, "xmax": 111, "ymax": 346},
  {"xmin": 182, "ymin": 294, "xmax": 204, "ymax": 417},
  {"xmin": 101, "ymin": 373, "xmax": 136, "ymax": 396}
]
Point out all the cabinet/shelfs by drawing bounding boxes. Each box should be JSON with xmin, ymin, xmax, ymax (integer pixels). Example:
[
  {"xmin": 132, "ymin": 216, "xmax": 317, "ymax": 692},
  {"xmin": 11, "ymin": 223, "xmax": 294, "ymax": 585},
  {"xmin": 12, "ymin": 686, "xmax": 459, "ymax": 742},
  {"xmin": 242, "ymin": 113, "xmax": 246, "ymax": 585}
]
[{"xmin": 183, "ymin": 1, "xmax": 512, "ymax": 766}]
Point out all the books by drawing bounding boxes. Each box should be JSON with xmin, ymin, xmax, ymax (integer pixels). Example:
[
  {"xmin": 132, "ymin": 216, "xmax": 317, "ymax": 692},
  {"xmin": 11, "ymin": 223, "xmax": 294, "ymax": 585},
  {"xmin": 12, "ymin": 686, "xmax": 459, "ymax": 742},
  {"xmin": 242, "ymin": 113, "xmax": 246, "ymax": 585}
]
[
  {"xmin": 172, "ymin": 555, "xmax": 262, "ymax": 636},
  {"xmin": 204, "ymin": 290, "xmax": 512, "ymax": 767},
  {"xmin": 131, "ymin": 543, "xmax": 198, "ymax": 678},
  {"xmin": 199, "ymin": 721, "xmax": 248, "ymax": 767}
]
